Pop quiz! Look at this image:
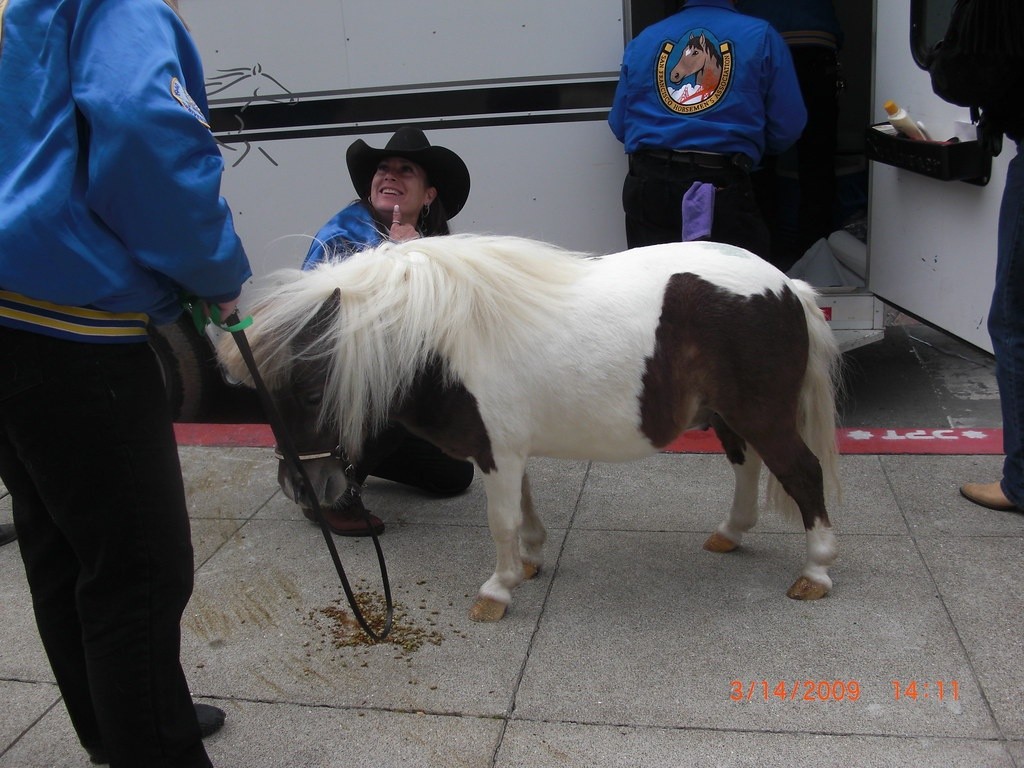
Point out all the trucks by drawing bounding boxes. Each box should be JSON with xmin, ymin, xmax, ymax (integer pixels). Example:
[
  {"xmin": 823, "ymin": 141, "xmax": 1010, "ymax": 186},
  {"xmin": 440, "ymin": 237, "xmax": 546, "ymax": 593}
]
[{"xmin": 150, "ymin": 0, "xmax": 1016, "ymax": 425}]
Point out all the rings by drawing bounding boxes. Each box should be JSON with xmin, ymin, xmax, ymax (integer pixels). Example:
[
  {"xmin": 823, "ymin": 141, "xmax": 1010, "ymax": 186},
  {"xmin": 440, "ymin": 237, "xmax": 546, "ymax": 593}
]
[{"xmin": 392, "ymin": 221, "xmax": 401, "ymax": 225}]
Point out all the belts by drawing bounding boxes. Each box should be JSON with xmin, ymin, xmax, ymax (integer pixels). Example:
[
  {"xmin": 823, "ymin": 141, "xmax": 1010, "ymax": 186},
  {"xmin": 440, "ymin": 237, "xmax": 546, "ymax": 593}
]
[{"xmin": 640, "ymin": 145, "xmax": 746, "ymax": 170}]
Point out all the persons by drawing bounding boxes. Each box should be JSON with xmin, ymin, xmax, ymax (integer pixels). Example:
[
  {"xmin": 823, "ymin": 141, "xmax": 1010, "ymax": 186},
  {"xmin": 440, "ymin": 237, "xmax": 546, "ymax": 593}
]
[
  {"xmin": 733, "ymin": 0, "xmax": 846, "ymax": 245},
  {"xmin": 0, "ymin": 0, "xmax": 253, "ymax": 768},
  {"xmin": 608, "ymin": 0, "xmax": 809, "ymax": 251},
  {"xmin": 276, "ymin": 126, "xmax": 474, "ymax": 535},
  {"xmin": 932, "ymin": 0, "xmax": 1024, "ymax": 515}
]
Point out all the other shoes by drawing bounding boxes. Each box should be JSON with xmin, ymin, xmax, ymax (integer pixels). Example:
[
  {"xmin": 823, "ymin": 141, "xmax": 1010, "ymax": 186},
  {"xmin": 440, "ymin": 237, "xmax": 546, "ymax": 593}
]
[
  {"xmin": 300, "ymin": 502, "xmax": 385, "ymax": 537},
  {"xmin": 192, "ymin": 702, "xmax": 225, "ymax": 737},
  {"xmin": 960, "ymin": 481, "xmax": 1015, "ymax": 510}
]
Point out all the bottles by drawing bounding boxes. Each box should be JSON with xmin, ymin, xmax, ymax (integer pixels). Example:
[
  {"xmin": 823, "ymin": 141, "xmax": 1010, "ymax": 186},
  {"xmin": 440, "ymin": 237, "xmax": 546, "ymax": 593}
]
[{"xmin": 884, "ymin": 100, "xmax": 925, "ymax": 142}]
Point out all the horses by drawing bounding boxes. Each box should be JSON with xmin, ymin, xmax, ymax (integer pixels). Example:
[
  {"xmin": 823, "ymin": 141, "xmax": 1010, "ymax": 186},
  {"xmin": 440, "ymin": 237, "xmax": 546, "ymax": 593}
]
[{"xmin": 214, "ymin": 230, "xmax": 844, "ymax": 624}]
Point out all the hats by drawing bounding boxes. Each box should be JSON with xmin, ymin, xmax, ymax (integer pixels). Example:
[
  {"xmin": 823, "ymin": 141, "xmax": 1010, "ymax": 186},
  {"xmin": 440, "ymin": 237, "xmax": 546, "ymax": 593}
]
[{"xmin": 345, "ymin": 124, "xmax": 470, "ymax": 228}]
